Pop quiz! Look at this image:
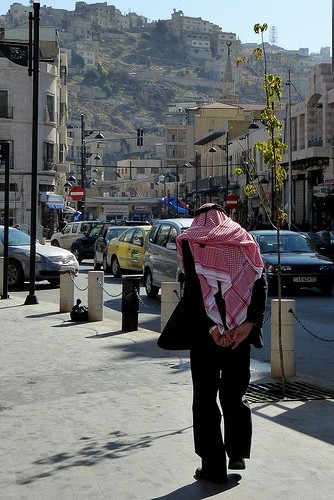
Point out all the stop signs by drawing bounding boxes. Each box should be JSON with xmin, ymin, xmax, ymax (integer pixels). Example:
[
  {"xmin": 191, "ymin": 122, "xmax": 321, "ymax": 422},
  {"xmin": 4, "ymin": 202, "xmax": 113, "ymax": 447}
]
[
  {"xmin": 69, "ymin": 185, "xmax": 84, "ymax": 201},
  {"xmin": 224, "ymin": 195, "xmax": 238, "ymax": 209}
]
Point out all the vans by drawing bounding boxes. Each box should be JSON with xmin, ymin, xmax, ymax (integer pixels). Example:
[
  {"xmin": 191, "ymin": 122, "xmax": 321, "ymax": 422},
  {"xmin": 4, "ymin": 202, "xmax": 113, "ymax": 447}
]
[{"xmin": 142, "ymin": 218, "xmax": 194, "ymax": 298}]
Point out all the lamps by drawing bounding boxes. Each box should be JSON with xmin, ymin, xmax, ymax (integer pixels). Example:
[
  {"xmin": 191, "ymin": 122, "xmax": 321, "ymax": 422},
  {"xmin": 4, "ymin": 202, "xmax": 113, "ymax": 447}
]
[{"xmin": 58, "ymin": 172, "xmax": 77, "ymax": 186}]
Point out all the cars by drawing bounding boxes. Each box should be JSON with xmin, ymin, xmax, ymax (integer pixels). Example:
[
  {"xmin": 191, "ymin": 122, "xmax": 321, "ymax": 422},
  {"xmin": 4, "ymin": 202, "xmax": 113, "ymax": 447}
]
[
  {"xmin": 93, "ymin": 225, "xmax": 152, "ymax": 278},
  {"xmin": 0, "ymin": 225, "xmax": 80, "ymax": 290},
  {"xmin": 249, "ymin": 230, "xmax": 334, "ymax": 296}
]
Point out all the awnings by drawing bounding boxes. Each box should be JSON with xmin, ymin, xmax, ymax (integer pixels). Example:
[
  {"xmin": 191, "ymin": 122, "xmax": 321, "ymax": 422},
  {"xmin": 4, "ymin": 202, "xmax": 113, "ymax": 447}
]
[{"xmin": 43, "ymin": 203, "xmax": 65, "ymax": 209}]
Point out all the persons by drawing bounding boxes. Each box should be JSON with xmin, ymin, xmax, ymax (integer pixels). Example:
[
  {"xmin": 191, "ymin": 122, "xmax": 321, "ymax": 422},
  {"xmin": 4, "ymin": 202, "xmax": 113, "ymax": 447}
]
[
  {"xmin": 157, "ymin": 203, "xmax": 268, "ymax": 483},
  {"xmin": 57, "ymin": 218, "xmax": 67, "ymax": 233},
  {"xmin": 14, "ymin": 224, "xmax": 21, "ymax": 230}
]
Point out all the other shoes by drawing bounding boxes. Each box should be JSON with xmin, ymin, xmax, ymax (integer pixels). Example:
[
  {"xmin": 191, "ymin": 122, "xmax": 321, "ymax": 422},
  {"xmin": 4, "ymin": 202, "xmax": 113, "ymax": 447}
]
[
  {"xmin": 196, "ymin": 466, "xmax": 230, "ymax": 485},
  {"xmin": 227, "ymin": 456, "xmax": 247, "ymax": 470}
]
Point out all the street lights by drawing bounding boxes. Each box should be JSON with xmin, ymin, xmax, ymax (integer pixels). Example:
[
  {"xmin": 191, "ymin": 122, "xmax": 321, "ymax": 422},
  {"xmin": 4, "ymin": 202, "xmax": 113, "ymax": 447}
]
[
  {"xmin": 155, "ymin": 163, "xmax": 179, "ymax": 219},
  {"xmin": 208, "ymin": 132, "xmax": 229, "ymax": 218},
  {"xmin": 182, "ymin": 151, "xmax": 198, "ymax": 208},
  {"xmin": 81, "ymin": 113, "xmax": 105, "ymax": 221},
  {"xmin": 248, "ymin": 102, "xmax": 275, "ymax": 229}
]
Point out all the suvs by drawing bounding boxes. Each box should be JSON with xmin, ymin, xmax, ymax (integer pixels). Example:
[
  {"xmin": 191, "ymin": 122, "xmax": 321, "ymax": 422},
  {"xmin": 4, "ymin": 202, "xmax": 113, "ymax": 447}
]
[{"xmin": 51, "ymin": 221, "xmax": 152, "ymax": 265}]
